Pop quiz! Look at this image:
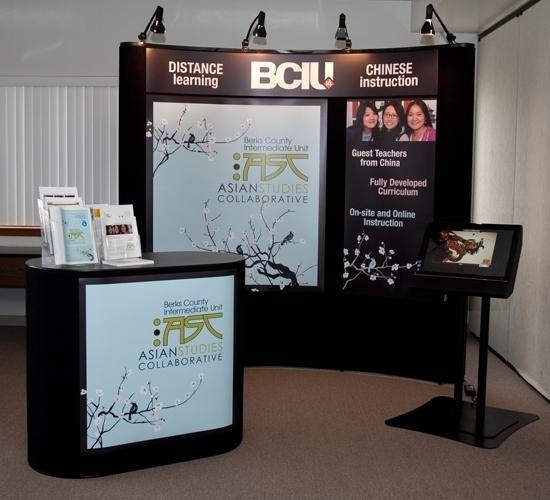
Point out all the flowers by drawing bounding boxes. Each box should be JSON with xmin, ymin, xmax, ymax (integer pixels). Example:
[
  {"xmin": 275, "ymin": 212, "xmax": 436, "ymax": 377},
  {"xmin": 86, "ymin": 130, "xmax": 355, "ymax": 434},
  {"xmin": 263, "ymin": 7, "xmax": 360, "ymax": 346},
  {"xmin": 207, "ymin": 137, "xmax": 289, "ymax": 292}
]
[
  {"xmin": 146, "ymin": 105, "xmax": 253, "ymax": 177},
  {"xmin": 80, "ymin": 365, "xmax": 205, "ymax": 450},
  {"xmin": 178, "ymin": 197, "xmax": 319, "ymax": 291},
  {"xmin": 342, "ymin": 230, "xmax": 423, "ymax": 292}
]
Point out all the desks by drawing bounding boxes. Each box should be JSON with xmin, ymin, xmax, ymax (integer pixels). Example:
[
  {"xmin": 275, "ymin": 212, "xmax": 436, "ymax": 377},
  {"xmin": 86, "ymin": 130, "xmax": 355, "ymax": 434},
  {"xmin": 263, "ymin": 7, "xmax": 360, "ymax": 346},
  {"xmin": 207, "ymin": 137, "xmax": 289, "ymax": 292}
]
[
  {"xmin": 24, "ymin": 249, "xmax": 246, "ymax": 480},
  {"xmin": 0, "ymin": 245, "xmax": 42, "ymax": 288}
]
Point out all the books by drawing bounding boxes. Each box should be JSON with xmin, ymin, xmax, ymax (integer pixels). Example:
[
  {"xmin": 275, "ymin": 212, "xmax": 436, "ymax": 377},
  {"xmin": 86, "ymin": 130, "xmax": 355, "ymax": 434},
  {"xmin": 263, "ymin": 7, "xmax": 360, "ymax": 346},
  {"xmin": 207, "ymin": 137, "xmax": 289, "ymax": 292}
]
[{"xmin": 38, "ymin": 185, "xmax": 154, "ymax": 267}]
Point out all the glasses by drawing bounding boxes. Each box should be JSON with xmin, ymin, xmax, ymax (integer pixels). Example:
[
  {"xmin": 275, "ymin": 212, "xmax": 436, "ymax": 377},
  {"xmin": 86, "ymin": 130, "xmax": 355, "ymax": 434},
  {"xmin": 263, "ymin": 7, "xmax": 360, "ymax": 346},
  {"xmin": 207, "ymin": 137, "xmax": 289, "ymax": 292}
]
[{"xmin": 384, "ymin": 113, "xmax": 398, "ymax": 119}]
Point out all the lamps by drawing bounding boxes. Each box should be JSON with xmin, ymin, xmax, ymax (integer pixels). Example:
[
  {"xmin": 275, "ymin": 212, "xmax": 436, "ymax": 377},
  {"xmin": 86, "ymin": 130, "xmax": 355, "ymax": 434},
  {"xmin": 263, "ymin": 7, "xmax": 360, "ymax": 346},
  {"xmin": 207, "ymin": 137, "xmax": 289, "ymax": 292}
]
[
  {"xmin": 138, "ymin": 5, "xmax": 165, "ymax": 42},
  {"xmin": 420, "ymin": 3, "xmax": 457, "ymax": 44},
  {"xmin": 335, "ymin": 13, "xmax": 353, "ymax": 49},
  {"xmin": 242, "ymin": 10, "xmax": 266, "ymax": 53}
]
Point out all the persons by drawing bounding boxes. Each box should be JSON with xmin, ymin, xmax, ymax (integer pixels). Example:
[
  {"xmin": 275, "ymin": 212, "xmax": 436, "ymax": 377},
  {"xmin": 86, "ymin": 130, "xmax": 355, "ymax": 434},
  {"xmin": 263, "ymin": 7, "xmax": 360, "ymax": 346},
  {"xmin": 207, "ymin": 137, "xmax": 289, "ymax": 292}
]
[
  {"xmin": 379, "ymin": 101, "xmax": 411, "ymax": 142},
  {"xmin": 431, "ymin": 230, "xmax": 484, "ymax": 266},
  {"xmin": 347, "ymin": 102, "xmax": 382, "ymax": 143},
  {"xmin": 404, "ymin": 99, "xmax": 436, "ymax": 142}
]
